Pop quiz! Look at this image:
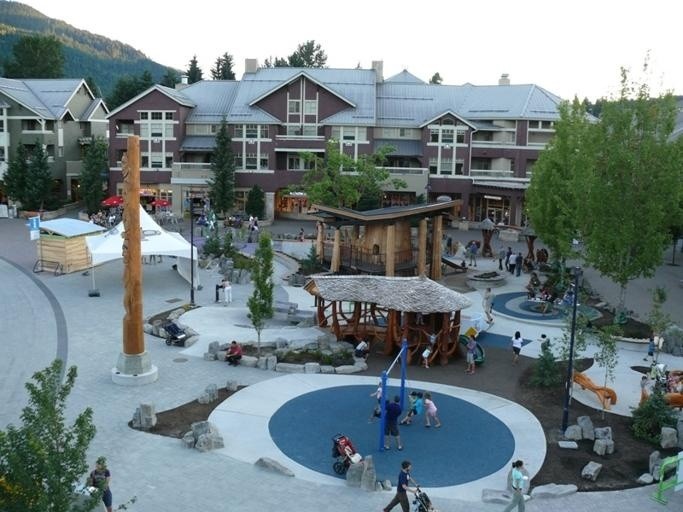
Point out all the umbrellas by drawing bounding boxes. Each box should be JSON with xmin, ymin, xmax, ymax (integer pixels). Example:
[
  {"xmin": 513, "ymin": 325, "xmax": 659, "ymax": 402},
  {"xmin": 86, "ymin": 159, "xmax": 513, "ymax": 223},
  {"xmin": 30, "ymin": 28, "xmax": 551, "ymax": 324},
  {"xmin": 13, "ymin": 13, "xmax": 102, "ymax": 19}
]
[
  {"xmin": 101, "ymin": 196, "xmax": 124, "ymax": 207},
  {"xmin": 150, "ymin": 199, "xmax": 170, "ymax": 213}
]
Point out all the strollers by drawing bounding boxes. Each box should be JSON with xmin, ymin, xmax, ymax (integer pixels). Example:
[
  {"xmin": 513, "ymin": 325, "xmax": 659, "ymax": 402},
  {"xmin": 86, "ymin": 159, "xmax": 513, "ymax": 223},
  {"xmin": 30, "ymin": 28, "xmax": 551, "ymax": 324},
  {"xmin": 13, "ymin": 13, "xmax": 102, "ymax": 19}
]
[
  {"xmin": 646, "ymin": 363, "xmax": 667, "ymax": 391},
  {"xmin": 414, "ymin": 484, "xmax": 437, "ymax": 512},
  {"xmin": 161, "ymin": 319, "xmax": 186, "ymax": 348},
  {"xmin": 330, "ymin": 429, "xmax": 361, "ymax": 477}
]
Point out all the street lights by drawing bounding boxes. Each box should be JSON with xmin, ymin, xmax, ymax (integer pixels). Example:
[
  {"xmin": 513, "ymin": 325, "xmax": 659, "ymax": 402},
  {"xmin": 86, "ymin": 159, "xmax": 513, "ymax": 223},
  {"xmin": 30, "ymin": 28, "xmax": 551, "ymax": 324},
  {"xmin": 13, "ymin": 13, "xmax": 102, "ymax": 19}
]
[
  {"xmin": 186, "ymin": 196, "xmax": 210, "ymax": 309},
  {"xmin": 558, "ymin": 266, "xmax": 585, "ymax": 434}
]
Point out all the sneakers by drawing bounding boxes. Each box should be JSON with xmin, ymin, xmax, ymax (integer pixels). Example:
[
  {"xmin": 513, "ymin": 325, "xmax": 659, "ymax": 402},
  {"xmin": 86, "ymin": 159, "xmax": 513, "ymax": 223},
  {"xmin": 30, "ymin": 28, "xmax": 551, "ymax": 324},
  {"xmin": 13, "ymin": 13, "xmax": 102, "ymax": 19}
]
[{"xmin": 383, "ymin": 444, "xmax": 404, "ymax": 451}]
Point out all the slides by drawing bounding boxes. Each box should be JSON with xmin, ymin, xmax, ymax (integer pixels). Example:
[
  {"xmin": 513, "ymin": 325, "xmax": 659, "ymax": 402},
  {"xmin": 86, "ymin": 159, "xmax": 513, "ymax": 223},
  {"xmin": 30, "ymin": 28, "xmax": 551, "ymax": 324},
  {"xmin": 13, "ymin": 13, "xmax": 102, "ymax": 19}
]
[
  {"xmin": 426, "ymin": 237, "xmax": 469, "ymax": 270},
  {"xmin": 459, "ymin": 335, "xmax": 484, "ymax": 364}
]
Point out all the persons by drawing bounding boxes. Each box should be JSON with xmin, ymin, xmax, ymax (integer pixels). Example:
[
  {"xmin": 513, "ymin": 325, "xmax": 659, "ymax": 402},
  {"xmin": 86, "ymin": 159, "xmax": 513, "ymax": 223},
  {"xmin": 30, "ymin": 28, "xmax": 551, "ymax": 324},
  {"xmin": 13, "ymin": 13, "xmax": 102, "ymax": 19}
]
[
  {"xmin": 368, "ymin": 380, "xmax": 442, "ymax": 452},
  {"xmin": 463, "ymin": 336, "xmax": 477, "ymax": 375},
  {"xmin": 511, "ymin": 331, "xmax": 524, "ymax": 366},
  {"xmin": 196, "ymin": 196, "xmax": 259, "ymax": 235},
  {"xmin": 14, "ymin": 199, "xmax": 22, "ymax": 218},
  {"xmin": 539, "ymin": 334, "xmax": 550, "ymax": 355},
  {"xmin": 430, "ymin": 333, "xmax": 438, "ymax": 347},
  {"xmin": 7, "ymin": 197, "xmax": 14, "ymax": 217},
  {"xmin": 503, "ymin": 460, "xmax": 528, "ymax": 512},
  {"xmin": 299, "ymin": 228, "xmax": 305, "ymax": 241},
  {"xmin": 496, "ymin": 244, "xmax": 575, "ymax": 313},
  {"xmin": 222, "ymin": 279, "xmax": 232, "ymax": 306},
  {"xmin": 469, "ymin": 241, "xmax": 478, "ymax": 267},
  {"xmin": 421, "ymin": 345, "xmax": 433, "ymax": 368},
  {"xmin": 141, "ymin": 256, "xmax": 164, "ymax": 266},
  {"xmin": 356, "ymin": 338, "xmax": 367, "ymax": 358},
  {"xmin": 383, "ymin": 461, "xmax": 418, "ymax": 511},
  {"xmin": 639, "ymin": 330, "xmax": 683, "ymax": 404},
  {"xmin": 91, "ymin": 204, "xmax": 178, "ymax": 231},
  {"xmin": 225, "ymin": 341, "xmax": 243, "ymax": 366},
  {"xmin": 483, "ymin": 287, "xmax": 495, "ymax": 324}
]
